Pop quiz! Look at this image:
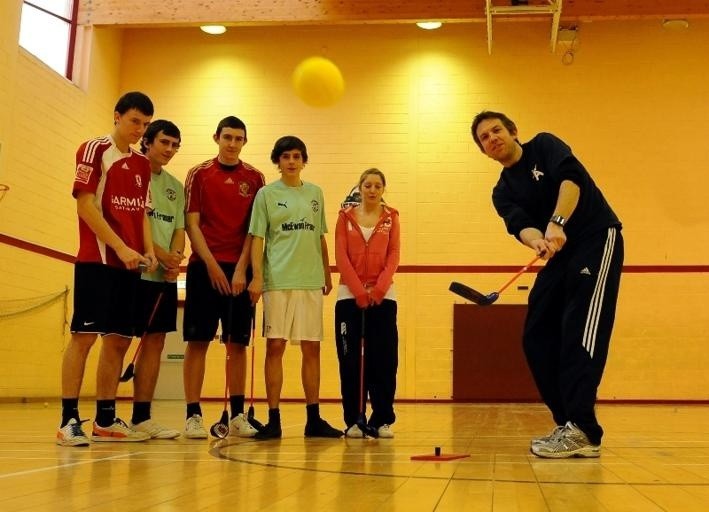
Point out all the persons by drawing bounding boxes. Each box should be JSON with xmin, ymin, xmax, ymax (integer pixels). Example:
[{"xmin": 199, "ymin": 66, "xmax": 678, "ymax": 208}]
[
  {"xmin": 184, "ymin": 115, "xmax": 264, "ymax": 439},
  {"xmin": 55, "ymin": 90, "xmax": 154, "ymax": 450},
  {"xmin": 142, "ymin": 119, "xmax": 187, "ymax": 442},
  {"xmin": 249, "ymin": 134, "xmax": 345, "ymax": 439},
  {"xmin": 470, "ymin": 111, "xmax": 625, "ymax": 460},
  {"xmin": 333, "ymin": 166, "xmax": 399, "ymax": 439}
]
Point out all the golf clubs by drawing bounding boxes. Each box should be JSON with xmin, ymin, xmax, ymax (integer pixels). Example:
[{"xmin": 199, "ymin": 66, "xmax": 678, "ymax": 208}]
[
  {"xmin": 356, "ymin": 312, "xmax": 380, "ymax": 438},
  {"xmin": 118, "ymin": 281, "xmax": 171, "ymax": 384},
  {"xmin": 449, "ymin": 252, "xmax": 548, "ymax": 307},
  {"xmin": 211, "ymin": 292, "xmax": 233, "ymax": 438},
  {"xmin": 247, "ymin": 296, "xmax": 273, "ymax": 432}
]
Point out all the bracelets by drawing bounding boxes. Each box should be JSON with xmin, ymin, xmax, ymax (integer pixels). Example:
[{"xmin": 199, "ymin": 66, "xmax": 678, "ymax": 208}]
[{"xmin": 548, "ymin": 214, "xmax": 567, "ymax": 227}]
